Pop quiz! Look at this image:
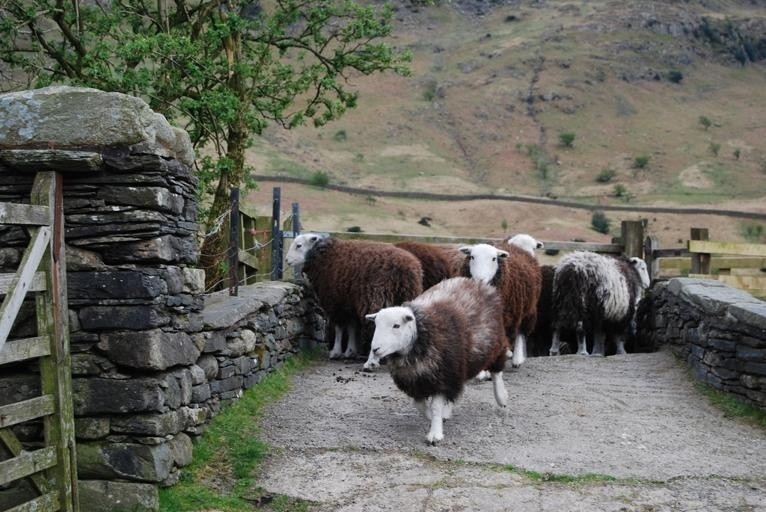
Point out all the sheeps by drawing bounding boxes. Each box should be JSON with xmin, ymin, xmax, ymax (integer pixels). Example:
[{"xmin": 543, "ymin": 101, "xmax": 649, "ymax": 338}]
[
  {"xmin": 285, "ymin": 232, "xmax": 651, "ymax": 382},
  {"xmin": 364, "ymin": 275, "xmax": 512, "ymax": 444}
]
